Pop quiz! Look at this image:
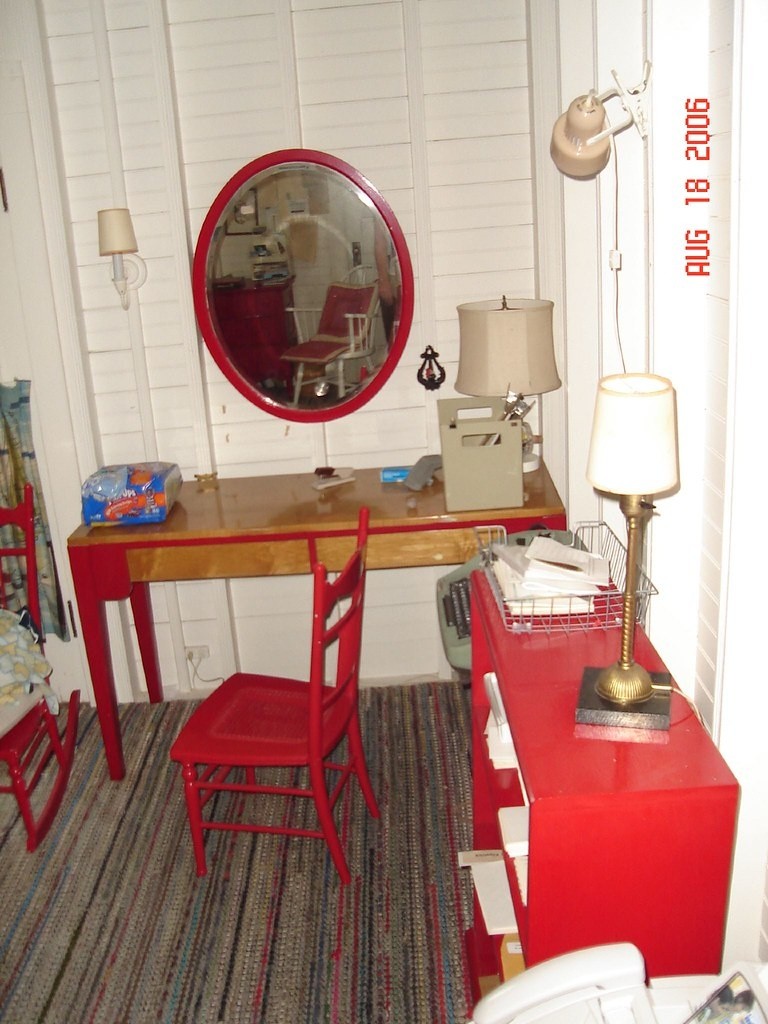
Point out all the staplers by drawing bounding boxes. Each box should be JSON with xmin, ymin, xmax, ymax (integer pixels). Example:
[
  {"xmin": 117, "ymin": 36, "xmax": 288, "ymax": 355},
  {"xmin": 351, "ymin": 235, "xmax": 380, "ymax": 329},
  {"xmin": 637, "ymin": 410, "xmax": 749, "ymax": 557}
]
[{"xmin": 310, "ymin": 466, "xmax": 357, "ymax": 490}]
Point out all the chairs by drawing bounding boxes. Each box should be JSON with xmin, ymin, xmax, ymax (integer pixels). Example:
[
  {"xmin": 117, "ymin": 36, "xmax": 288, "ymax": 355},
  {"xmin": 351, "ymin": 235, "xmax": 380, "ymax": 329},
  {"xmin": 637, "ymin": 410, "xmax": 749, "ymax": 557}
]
[
  {"xmin": 0, "ymin": 483, "xmax": 81, "ymax": 854},
  {"xmin": 170, "ymin": 508, "xmax": 381, "ymax": 885},
  {"xmin": 280, "ymin": 263, "xmax": 381, "ymax": 405}
]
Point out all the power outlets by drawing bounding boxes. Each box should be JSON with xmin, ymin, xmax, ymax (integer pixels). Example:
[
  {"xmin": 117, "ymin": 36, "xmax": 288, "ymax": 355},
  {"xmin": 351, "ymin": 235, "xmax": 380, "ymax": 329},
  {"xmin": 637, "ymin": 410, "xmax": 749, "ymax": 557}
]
[{"xmin": 184, "ymin": 646, "xmax": 209, "ymax": 660}]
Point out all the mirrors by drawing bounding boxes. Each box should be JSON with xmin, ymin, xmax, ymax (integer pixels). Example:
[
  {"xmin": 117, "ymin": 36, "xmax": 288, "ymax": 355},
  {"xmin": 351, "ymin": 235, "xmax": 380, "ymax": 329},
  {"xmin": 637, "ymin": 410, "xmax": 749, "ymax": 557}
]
[{"xmin": 191, "ymin": 149, "xmax": 414, "ymax": 423}]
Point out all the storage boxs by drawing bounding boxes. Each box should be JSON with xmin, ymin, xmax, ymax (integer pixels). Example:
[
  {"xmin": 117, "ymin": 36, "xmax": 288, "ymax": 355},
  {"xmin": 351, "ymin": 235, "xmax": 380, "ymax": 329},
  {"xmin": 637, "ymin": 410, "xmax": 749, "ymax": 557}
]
[
  {"xmin": 501, "ymin": 934, "xmax": 526, "ymax": 983},
  {"xmin": 382, "ymin": 467, "xmax": 410, "ymax": 483}
]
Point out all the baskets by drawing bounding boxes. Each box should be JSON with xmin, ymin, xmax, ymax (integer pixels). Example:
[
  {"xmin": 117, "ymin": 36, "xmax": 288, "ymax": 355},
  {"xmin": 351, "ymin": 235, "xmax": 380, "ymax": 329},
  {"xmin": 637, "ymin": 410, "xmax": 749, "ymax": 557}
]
[{"xmin": 474, "ymin": 520, "xmax": 658, "ymax": 636}]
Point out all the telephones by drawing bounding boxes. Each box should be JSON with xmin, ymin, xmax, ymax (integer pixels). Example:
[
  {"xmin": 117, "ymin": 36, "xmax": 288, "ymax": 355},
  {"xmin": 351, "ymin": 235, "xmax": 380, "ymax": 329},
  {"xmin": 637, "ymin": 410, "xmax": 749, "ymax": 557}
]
[{"xmin": 472, "ymin": 941, "xmax": 658, "ymax": 1024}]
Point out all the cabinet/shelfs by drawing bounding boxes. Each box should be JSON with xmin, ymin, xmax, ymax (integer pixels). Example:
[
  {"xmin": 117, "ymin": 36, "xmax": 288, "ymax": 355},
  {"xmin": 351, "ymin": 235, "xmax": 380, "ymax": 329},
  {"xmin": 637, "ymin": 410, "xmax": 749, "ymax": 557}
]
[
  {"xmin": 214, "ymin": 275, "xmax": 298, "ymax": 403},
  {"xmin": 463, "ymin": 568, "xmax": 742, "ymax": 1005}
]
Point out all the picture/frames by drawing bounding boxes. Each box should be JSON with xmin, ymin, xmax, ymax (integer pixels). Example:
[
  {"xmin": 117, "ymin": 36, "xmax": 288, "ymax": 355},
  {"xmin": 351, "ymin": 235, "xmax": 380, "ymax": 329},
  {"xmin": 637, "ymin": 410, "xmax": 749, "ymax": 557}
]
[{"xmin": 224, "ymin": 188, "xmax": 259, "ymax": 235}]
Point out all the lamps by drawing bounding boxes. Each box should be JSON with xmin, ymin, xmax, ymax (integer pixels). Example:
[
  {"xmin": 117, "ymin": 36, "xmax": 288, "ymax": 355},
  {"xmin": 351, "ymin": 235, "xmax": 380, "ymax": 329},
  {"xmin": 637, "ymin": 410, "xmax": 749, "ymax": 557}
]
[
  {"xmin": 98, "ymin": 208, "xmax": 147, "ymax": 312},
  {"xmin": 454, "ymin": 294, "xmax": 563, "ymax": 474},
  {"xmin": 549, "ymin": 88, "xmax": 634, "ymax": 177},
  {"xmin": 575, "ymin": 376, "xmax": 680, "ymax": 728}
]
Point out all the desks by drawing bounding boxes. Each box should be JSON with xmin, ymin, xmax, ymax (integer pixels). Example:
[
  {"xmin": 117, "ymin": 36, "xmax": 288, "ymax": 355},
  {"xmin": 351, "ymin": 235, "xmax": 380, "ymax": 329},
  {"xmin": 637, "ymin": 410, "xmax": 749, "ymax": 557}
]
[{"xmin": 67, "ymin": 458, "xmax": 568, "ymax": 780}]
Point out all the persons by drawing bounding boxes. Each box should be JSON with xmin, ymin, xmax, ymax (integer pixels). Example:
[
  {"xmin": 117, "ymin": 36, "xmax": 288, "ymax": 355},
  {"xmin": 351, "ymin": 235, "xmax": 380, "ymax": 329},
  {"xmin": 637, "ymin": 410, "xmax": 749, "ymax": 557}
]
[{"xmin": 373, "ymin": 216, "xmax": 403, "ymax": 351}]
